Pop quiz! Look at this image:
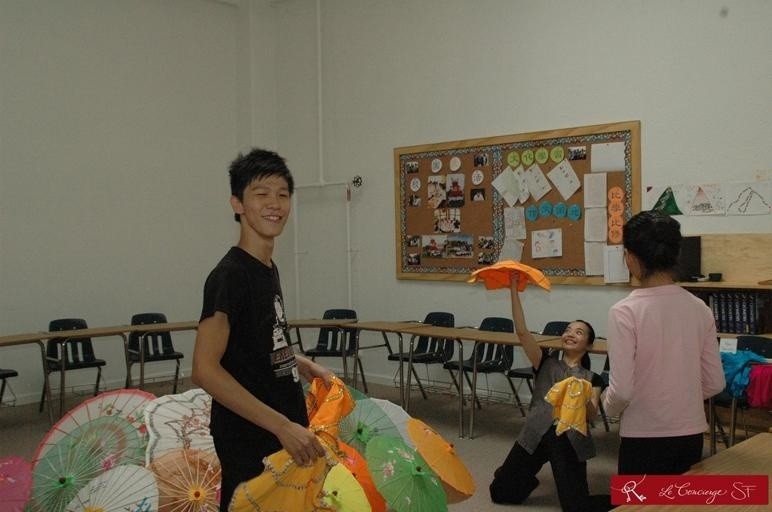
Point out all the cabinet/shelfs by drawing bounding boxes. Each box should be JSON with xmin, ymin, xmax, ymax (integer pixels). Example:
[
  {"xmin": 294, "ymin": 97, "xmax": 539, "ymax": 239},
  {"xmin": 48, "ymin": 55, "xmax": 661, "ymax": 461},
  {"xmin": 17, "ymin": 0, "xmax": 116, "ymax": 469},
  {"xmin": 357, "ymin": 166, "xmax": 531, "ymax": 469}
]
[{"xmin": 676, "ymin": 282, "xmax": 772, "ymax": 440}]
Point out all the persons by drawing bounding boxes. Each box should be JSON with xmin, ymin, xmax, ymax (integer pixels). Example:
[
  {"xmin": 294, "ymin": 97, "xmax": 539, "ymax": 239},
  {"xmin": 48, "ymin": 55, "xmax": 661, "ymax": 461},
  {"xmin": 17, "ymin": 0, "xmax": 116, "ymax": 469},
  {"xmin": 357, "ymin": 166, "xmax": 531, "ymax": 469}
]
[
  {"xmin": 594, "ymin": 211, "xmax": 725, "ymax": 504},
  {"xmin": 489, "ymin": 270, "xmax": 617, "ymax": 512},
  {"xmin": 189, "ymin": 146, "xmax": 335, "ymax": 511}
]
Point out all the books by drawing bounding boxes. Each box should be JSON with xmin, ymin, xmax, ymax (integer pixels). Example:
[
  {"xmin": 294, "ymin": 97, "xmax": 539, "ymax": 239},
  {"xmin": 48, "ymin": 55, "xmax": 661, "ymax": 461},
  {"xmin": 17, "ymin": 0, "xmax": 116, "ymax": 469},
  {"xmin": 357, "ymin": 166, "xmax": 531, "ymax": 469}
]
[{"xmin": 694, "ymin": 289, "xmax": 771, "ymax": 335}]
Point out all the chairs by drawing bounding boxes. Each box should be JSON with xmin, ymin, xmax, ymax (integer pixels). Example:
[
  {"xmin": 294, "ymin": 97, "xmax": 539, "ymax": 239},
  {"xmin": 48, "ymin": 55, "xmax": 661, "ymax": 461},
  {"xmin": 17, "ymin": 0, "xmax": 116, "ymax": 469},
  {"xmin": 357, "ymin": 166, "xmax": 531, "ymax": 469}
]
[
  {"xmin": 125, "ymin": 313, "xmax": 184, "ymax": 394},
  {"xmin": 508, "ymin": 321, "xmax": 595, "ymax": 433},
  {"xmin": 305, "ymin": 309, "xmax": 368, "ymax": 394},
  {"xmin": 599, "ymin": 354, "xmax": 610, "ymax": 431},
  {"xmin": 40, "ymin": 319, "xmax": 106, "ymax": 413},
  {"xmin": 443, "ymin": 317, "xmax": 526, "ymax": 417},
  {"xmin": 388, "ymin": 312, "xmax": 467, "ymax": 407},
  {"xmin": 0, "ymin": 369, "xmax": 18, "ymax": 406},
  {"xmin": 704, "ymin": 336, "xmax": 772, "ymax": 448}
]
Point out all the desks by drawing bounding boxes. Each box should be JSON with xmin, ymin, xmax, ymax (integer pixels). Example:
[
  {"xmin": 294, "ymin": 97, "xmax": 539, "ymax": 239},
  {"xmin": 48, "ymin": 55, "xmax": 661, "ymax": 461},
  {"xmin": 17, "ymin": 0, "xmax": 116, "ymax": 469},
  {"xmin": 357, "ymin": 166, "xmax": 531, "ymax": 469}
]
[
  {"xmin": 338, "ymin": 321, "xmax": 433, "ymax": 410},
  {"xmin": 40, "ymin": 325, "xmax": 133, "ymax": 418},
  {"xmin": 468, "ymin": 331, "xmax": 562, "ymax": 440},
  {"xmin": 288, "ymin": 319, "xmax": 358, "ymax": 384},
  {"xmin": 396, "ymin": 325, "xmax": 484, "ymax": 439},
  {"xmin": 536, "ymin": 339, "xmax": 608, "ymax": 355},
  {"xmin": 0, "ymin": 333, "xmax": 55, "ymax": 427},
  {"xmin": 608, "ymin": 432, "xmax": 772, "ymax": 512},
  {"xmin": 128, "ymin": 321, "xmax": 200, "ymax": 391}
]
